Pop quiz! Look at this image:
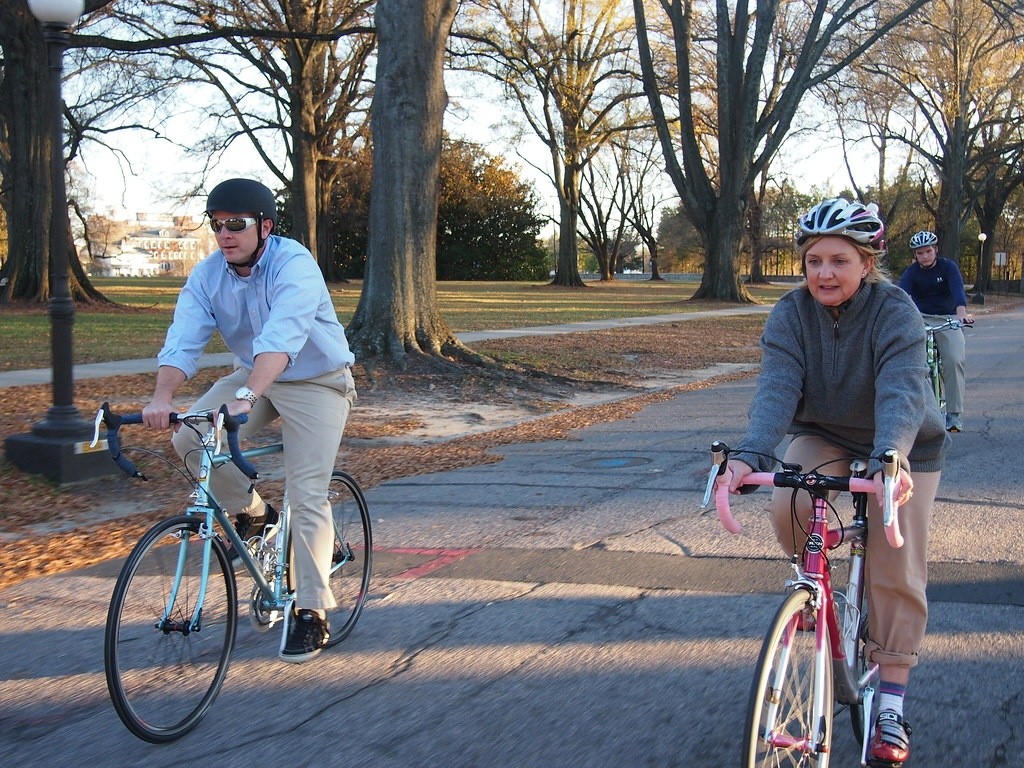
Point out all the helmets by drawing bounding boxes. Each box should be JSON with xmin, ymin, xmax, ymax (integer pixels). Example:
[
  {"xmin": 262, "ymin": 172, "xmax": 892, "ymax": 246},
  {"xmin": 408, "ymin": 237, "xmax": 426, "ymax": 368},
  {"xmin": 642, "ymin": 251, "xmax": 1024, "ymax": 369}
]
[
  {"xmin": 798, "ymin": 197, "xmax": 886, "ymax": 255},
  {"xmin": 206, "ymin": 178, "xmax": 277, "ymax": 235},
  {"xmin": 909, "ymin": 231, "xmax": 937, "ymax": 250}
]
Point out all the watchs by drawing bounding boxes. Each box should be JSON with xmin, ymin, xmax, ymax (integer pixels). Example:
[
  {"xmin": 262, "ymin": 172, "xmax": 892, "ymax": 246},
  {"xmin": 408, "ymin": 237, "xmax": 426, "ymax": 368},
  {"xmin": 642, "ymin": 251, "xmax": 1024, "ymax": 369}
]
[{"xmin": 235, "ymin": 386, "xmax": 257, "ymax": 407}]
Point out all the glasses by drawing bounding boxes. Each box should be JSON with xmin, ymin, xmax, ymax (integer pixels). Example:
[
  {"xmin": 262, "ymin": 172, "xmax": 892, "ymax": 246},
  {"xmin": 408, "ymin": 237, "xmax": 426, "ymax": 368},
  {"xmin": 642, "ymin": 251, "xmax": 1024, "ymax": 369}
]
[{"xmin": 208, "ymin": 218, "xmax": 257, "ymax": 233}]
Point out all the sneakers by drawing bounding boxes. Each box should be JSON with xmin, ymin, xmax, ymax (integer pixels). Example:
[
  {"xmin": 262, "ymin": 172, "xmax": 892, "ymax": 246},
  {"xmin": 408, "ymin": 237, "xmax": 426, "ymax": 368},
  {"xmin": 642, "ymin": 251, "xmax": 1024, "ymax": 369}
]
[
  {"xmin": 945, "ymin": 413, "xmax": 963, "ymax": 433},
  {"xmin": 793, "ymin": 609, "xmax": 818, "ymax": 633},
  {"xmin": 280, "ymin": 608, "xmax": 332, "ymax": 663},
  {"xmin": 870, "ymin": 708, "xmax": 912, "ymax": 762},
  {"xmin": 228, "ymin": 501, "xmax": 282, "ymax": 567}
]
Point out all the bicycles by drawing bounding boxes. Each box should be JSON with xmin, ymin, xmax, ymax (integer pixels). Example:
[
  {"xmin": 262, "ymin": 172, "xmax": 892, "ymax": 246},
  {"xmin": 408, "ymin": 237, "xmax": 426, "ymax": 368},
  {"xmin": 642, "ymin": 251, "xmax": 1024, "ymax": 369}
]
[
  {"xmin": 90, "ymin": 402, "xmax": 373, "ymax": 744},
  {"xmin": 923, "ymin": 317, "xmax": 978, "ymax": 432},
  {"xmin": 699, "ymin": 440, "xmax": 911, "ymax": 768}
]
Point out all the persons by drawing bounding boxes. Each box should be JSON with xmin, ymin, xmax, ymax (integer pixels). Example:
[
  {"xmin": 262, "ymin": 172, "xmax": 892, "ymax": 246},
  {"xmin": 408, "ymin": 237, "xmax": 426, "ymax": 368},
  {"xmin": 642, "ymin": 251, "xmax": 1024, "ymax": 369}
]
[
  {"xmin": 142, "ymin": 178, "xmax": 358, "ymax": 663},
  {"xmin": 897, "ymin": 230, "xmax": 974, "ymax": 433},
  {"xmin": 709, "ymin": 198, "xmax": 952, "ymax": 763}
]
